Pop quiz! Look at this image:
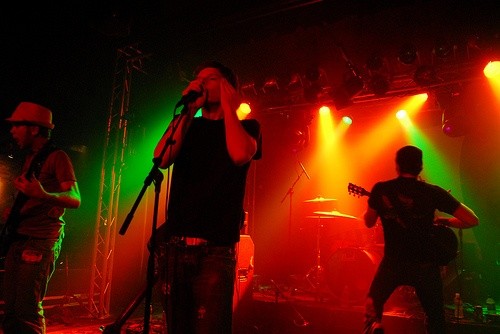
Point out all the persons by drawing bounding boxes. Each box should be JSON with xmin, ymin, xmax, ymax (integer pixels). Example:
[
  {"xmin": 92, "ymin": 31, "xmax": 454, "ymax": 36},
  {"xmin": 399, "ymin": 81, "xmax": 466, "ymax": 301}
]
[
  {"xmin": 153, "ymin": 63, "xmax": 261, "ymax": 334},
  {"xmin": 0, "ymin": 102, "xmax": 81, "ymax": 334},
  {"xmin": 363, "ymin": 146, "xmax": 479, "ymax": 334}
]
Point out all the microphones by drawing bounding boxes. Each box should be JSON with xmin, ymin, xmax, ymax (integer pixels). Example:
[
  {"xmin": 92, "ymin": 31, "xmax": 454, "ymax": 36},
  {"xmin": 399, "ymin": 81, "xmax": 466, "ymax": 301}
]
[
  {"xmin": 303, "ymin": 322, "xmax": 313, "ymax": 327},
  {"xmin": 176, "ymin": 91, "xmax": 203, "ymax": 107},
  {"xmin": 298, "ymin": 161, "xmax": 310, "ymax": 180}
]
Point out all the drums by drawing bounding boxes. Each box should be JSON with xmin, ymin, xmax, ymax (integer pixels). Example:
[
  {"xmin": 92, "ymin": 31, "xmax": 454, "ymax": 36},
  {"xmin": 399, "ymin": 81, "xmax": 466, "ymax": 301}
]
[{"xmin": 324, "ymin": 247, "xmax": 376, "ymax": 279}]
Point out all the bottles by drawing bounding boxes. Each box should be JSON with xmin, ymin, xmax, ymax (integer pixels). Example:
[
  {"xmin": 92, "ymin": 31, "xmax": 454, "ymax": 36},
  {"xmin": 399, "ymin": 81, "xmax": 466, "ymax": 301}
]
[
  {"xmin": 485, "ymin": 295, "xmax": 496, "ymax": 314},
  {"xmin": 454, "ymin": 293, "xmax": 463, "ymax": 320}
]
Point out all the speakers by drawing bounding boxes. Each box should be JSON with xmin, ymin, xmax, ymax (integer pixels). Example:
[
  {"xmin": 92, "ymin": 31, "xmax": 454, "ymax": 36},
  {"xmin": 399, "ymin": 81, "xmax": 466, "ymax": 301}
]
[{"xmin": 231, "ymin": 234, "xmax": 254, "ymax": 334}]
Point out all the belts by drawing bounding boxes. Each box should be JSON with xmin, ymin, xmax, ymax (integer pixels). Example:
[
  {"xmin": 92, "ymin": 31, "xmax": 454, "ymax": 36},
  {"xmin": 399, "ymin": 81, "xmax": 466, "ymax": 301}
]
[
  {"xmin": 176, "ymin": 246, "xmax": 233, "ymax": 257},
  {"xmin": 5, "ymin": 233, "xmax": 45, "ymax": 241}
]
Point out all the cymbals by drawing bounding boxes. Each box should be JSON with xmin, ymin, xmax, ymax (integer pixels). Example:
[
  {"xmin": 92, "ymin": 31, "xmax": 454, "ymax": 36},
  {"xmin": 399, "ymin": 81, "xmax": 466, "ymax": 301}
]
[
  {"xmin": 304, "ymin": 198, "xmax": 338, "ymax": 204},
  {"xmin": 313, "ymin": 210, "xmax": 358, "ymax": 220},
  {"xmin": 304, "ymin": 216, "xmax": 338, "ymax": 219}
]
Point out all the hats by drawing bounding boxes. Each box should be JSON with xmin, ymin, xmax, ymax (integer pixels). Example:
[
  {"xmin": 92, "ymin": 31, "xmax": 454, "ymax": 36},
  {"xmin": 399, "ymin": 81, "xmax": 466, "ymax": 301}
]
[{"xmin": 7, "ymin": 101, "xmax": 54, "ymax": 130}]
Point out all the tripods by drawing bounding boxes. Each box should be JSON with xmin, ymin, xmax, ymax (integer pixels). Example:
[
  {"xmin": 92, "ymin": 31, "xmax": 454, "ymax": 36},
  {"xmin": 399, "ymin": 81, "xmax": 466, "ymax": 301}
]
[{"xmin": 302, "ymin": 213, "xmax": 326, "ymax": 291}]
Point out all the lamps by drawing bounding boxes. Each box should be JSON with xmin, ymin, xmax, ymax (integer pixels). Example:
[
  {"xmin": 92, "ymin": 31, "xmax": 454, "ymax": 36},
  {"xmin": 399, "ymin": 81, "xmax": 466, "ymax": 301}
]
[
  {"xmin": 262, "ymin": 74, "xmax": 284, "ymax": 96},
  {"xmin": 327, "ymin": 74, "xmax": 367, "ymax": 109},
  {"xmin": 285, "ymin": 71, "xmax": 307, "ymax": 95},
  {"xmin": 396, "ymin": 40, "xmax": 422, "ymax": 75},
  {"xmin": 428, "ymin": 38, "xmax": 460, "ymax": 64},
  {"xmin": 433, "ymin": 87, "xmax": 475, "ymax": 140},
  {"xmin": 240, "ymin": 75, "xmax": 259, "ymax": 98},
  {"xmin": 280, "ymin": 109, "xmax": 313, "ymax": 152},
  {"xmin": 362, "ymin": 51, "xmax": 390, "ymax": 78}
]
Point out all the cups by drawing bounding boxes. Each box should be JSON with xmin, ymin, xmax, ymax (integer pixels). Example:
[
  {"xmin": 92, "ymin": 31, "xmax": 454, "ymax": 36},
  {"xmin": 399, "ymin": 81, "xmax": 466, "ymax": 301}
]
[{"xmin": 474, "ymin": 305, "xmax": 482, "ymax": 322}]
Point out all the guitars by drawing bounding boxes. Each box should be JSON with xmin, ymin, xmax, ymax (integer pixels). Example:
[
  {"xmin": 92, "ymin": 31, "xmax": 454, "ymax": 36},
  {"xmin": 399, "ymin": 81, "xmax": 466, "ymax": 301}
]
[{"xmin": 348, "ymin": 183, "xmax": 459, "ymax": 277}]
